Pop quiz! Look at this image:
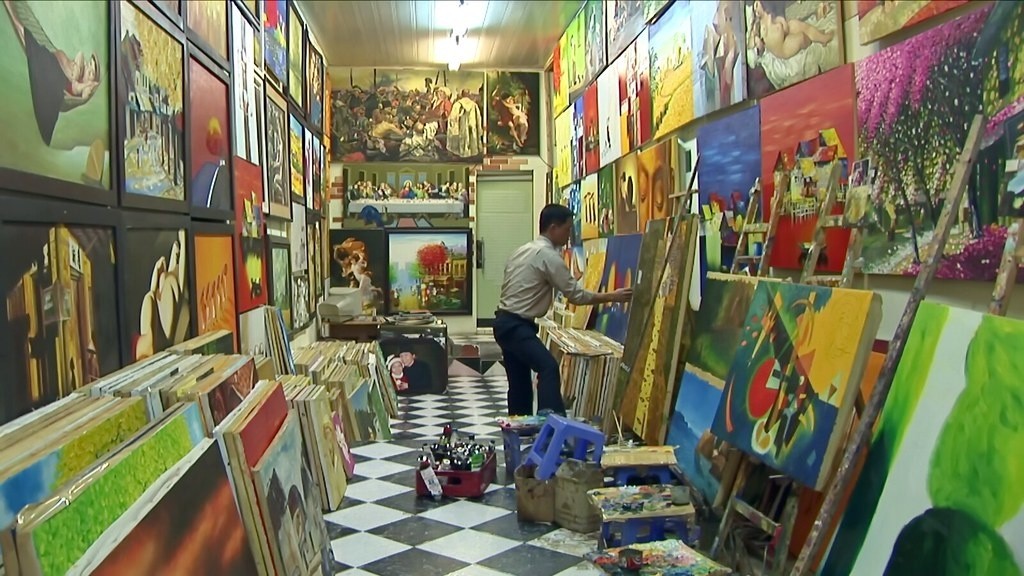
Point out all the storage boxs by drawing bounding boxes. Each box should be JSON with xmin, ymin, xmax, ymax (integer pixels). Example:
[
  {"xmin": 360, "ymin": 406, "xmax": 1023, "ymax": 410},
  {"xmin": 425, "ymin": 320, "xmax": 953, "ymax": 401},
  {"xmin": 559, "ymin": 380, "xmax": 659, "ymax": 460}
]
[{"xmin": 416, "ymin": 454, "xmax": 499, "ymax": 499}]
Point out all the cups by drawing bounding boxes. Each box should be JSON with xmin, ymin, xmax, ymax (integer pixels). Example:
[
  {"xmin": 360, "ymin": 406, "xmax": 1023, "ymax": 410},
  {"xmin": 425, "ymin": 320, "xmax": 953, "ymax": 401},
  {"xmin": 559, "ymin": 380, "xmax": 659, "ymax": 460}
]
[{"xmin": 670, "ymin": 486, "xmax": 690, "ymax": 506}]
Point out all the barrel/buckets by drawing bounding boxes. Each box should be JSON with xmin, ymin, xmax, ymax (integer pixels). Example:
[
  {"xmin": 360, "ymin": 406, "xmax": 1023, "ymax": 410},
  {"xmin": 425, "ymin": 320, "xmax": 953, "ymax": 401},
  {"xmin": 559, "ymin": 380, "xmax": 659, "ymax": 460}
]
[
  {"xmin": 553, "ymin": 457, "xmax": 604, "ymax": 532},
  {"xmin": 513, "ymin": 463, "xmax": 556, "ymax": 524}
]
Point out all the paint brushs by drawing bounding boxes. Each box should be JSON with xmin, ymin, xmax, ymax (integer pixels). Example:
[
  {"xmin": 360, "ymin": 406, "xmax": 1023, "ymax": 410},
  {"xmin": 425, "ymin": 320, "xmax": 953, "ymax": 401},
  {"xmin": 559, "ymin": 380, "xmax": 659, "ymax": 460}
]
[{"xmin": 613, "ymin": 410, "xmax": 624, "ymax": 445}]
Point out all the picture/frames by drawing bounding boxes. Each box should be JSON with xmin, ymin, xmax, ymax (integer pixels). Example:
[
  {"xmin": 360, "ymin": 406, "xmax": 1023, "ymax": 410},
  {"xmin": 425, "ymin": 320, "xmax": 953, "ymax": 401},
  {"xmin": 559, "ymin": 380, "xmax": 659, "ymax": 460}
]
[{"xmin": 0, "ymin": 0, "xmax": 474, "ymax": 426}]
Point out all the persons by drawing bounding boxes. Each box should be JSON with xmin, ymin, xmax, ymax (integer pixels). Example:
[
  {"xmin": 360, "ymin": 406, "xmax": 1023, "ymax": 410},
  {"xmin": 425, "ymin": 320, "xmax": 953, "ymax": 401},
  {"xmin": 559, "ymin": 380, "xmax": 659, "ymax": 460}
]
[
  {"xmin": 351, "ymin": 177, "xmax": 468, "ymax": 201},
  {"xmin": 385, "ymin": 344, "xmax": 432, "ymax": 395},
  {"xmin": 493, "ymin": 204, "xmax": 633, "ymax": 416},
  {"xmin": 330, "ymin": 78, "xmax": 482, "ymax": 160},
  {"xmin": 333, "ymin": 239, "xmax": 384, "ymax": 297},
  {"xmin": 492, "ymin": 85, "xmax": 528, "ymax": 147},
  {"xmin": 749, "ymin": 0, "xmax": 835, "ymax": 61},
  {"xmin": 3, "ymin": 1, "xmax": 100, "ymax": 100}
]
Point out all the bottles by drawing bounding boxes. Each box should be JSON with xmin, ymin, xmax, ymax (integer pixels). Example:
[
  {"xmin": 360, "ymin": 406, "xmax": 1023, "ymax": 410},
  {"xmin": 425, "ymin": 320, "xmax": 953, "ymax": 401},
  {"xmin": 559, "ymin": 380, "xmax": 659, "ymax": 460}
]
[{"xmin": 416, "ymin": 424, "xmax": 496, "ymax": 484}]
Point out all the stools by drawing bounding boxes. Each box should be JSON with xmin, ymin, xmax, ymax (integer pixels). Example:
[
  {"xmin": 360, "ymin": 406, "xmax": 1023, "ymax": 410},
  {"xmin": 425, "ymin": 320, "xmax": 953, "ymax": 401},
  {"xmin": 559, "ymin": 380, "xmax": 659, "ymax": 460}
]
[
  {"xmin": 499, "ymin": 429, "xmax": 557, "ymax": 478},
  {"xmin": 599, "ymin": 516, "xmax": 689, "ymax": 547},
  {"xmin": 618, "ymin": 466, "xmax": 676, "ymax": 535},
  {"xmin": 525, "ymin": 413, "xmax": 606, "ymax": 479}
]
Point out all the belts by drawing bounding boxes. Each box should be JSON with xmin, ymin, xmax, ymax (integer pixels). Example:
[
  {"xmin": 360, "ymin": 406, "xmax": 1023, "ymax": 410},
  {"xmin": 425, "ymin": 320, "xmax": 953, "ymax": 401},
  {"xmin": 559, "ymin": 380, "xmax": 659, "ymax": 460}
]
[{"xmin": 495, "ymin": 308, "xmax": 539, "ymax": 334}]
